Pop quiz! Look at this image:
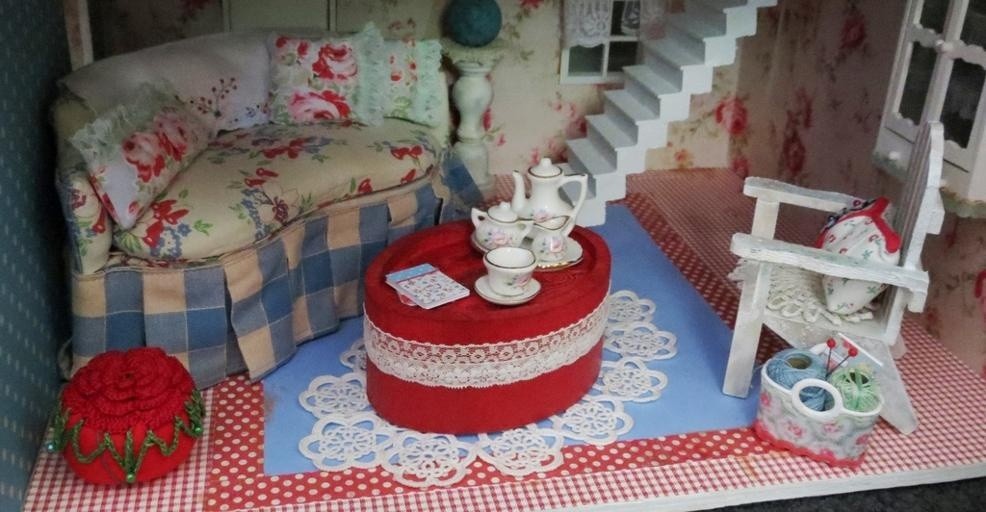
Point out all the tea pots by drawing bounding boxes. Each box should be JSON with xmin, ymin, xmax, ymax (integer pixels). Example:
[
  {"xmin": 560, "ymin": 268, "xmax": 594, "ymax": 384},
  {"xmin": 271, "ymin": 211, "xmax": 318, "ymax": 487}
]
[{"xmin": 510, "ymin": 157, "xmax": 588, "ymax": 241}]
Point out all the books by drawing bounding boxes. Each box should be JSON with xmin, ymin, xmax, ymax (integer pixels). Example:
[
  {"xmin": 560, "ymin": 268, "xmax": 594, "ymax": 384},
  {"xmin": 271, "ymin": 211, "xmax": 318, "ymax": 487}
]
[{"xmin": 385, "ymin": 263, "xmax": 470, "ymax": 310}]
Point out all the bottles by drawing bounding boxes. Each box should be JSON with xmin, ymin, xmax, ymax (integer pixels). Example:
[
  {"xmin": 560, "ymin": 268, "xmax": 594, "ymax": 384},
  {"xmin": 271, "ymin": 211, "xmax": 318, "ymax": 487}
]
[
  {"xmin": 531, "ymin": 214, "xmax": 573, "ymax": 261},
  {"xmin": 471, "ymin": 203, "xmax": 531, "ymax": 251}
]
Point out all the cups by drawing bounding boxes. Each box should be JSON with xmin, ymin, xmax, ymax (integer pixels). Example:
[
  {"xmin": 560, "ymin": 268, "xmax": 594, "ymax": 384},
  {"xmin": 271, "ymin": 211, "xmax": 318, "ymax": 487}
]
[{"xmin": 482, "ymin": 245, "xmax": 536, "ymax": 294}]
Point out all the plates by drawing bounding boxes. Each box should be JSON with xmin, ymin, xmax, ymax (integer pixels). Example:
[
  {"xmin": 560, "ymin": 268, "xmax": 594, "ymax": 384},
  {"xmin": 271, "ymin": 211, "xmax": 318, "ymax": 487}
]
[
  {"xmin": 470, "ymin": 228, "xmax": 585, "ymax": 272},
  {"xmin": 475, "ymin": 275, "xmax": 542, "ymax": 306}
]
